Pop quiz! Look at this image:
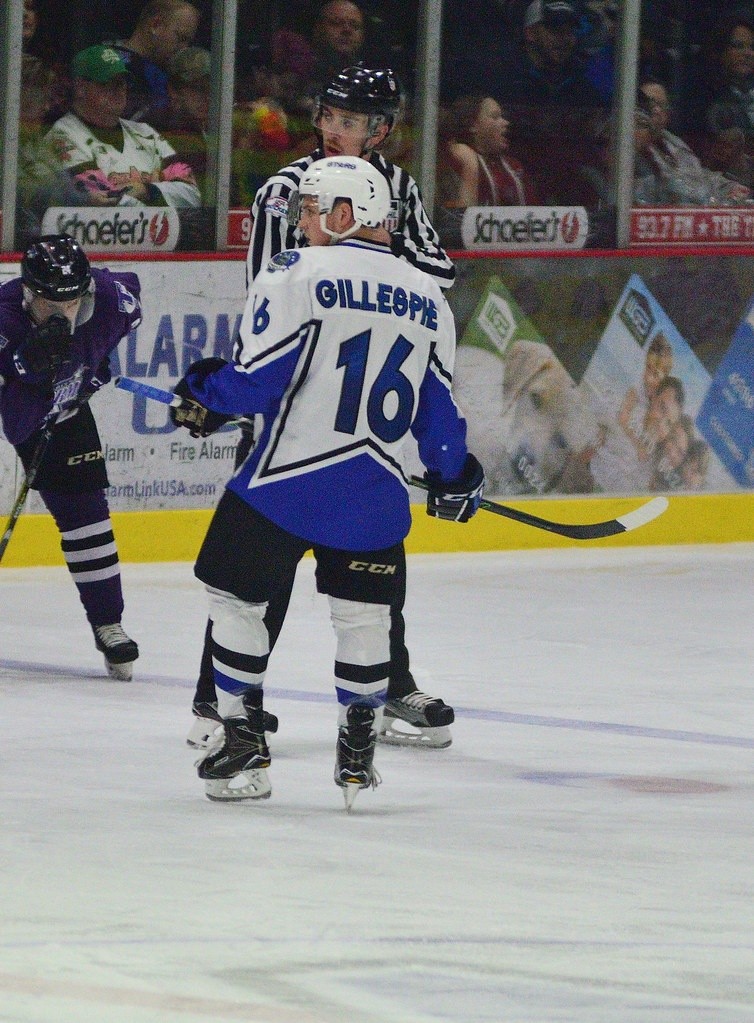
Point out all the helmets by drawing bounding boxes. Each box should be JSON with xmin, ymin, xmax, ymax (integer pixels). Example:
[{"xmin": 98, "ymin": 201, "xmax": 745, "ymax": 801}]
[
  {"xmin": 22, "ymin": 235, "xmax": 91, "ymax": 301},
  {"xmin": 323, "ymin": 60, "xmax": 401, "ymax": 113},
  {"xmin": 299, "ymin": 155, "xmax": 391, "ymax": 227}
]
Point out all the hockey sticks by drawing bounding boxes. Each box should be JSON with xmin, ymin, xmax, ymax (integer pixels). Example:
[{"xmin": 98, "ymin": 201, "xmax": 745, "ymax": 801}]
[
  {"xmin": 1, "ymin": 414, "xmax": 60, "ymax": 562},
  {"xmin": 111, "ymin": 373, "xmax": 673, "ymax": 542}
]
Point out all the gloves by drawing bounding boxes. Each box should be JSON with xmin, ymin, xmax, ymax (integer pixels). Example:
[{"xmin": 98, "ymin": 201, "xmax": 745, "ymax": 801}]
[
  {"xmin": 171, "ymin": 358, "xmax": 236, "ymax": 438},
  {"xmin": 12, "ymin": 314, "xmax": 71, "ymax": 378},
  {"xmin": 424, "ymin": 452, "xmax": 484, "ymax": 522}
]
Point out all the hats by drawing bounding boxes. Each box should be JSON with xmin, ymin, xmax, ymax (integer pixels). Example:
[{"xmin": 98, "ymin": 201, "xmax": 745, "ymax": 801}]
[
  {"xmin": 524, "ymin": 0, "xmax": 574, "ymax": 27},
  {"xmin": 71, "ymin": 46, "xmax": 135, "ymax": 84}
]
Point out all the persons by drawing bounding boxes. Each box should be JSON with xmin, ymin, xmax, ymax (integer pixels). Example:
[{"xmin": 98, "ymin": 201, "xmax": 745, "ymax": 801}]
[
  {"xmin": 0, "ymin": 0, "xmax": 754, "ymax": 216},
  {"xmin": 168, "ymin": 155, "xmax": 484, "ymax": 817},
  {"xmin": 569, "ymin": 331, "xmax": 708, "ymax": 497},
  {"xmin": 185, "ymin": 61, "xmax": 458, "ymax": 748},
  {"xmin": 0, "ymin": 233, "xmax": 143, "ymax": 681}
]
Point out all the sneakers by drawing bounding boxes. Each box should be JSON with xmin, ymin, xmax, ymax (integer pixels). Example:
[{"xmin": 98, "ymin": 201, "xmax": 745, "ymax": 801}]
[
  {"xmin": 93, "ymin": 623, "xmax": 141, "ymax": 681},
  {"xmin": 377, "ymin": 690, "xmax": 455, "ymax": 747},
  {"xmin": 186, "ymin": 698, "xmax": 279, "ymax": 748},
  {"xmin": 337, "ymin": 703, "xmax": 383, "ymax": 810},
  {"xmin": 197, "ymin": 690, "xmax": 273, "ymax": 801}
]
